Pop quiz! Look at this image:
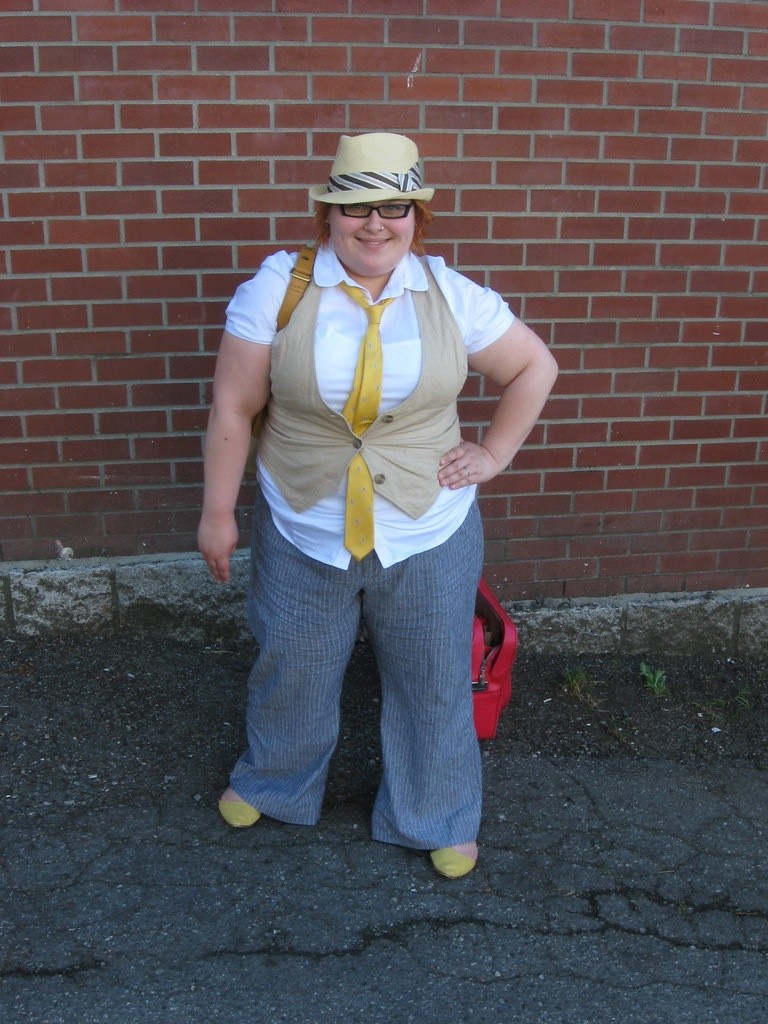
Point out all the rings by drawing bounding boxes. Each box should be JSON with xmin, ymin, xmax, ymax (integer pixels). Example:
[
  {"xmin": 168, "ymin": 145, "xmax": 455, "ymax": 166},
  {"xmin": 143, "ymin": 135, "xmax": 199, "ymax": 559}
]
[{"xmin": 464, "ymin": 467, "xmax": 470, "ymax": 476}]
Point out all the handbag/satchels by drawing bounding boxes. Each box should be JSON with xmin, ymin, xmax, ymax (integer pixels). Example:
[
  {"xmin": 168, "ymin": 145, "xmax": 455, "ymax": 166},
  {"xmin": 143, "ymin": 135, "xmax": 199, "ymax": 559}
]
[{"xmin": 470, "ymin": 577, "xmax": 518, "ymax": 738}]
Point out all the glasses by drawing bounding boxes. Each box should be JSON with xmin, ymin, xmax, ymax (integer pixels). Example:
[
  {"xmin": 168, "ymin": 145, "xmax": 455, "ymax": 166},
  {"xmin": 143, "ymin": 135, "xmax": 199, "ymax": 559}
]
[{"xmin": 337, "ymin": 202, "xmax": 414, "ymax": 219}]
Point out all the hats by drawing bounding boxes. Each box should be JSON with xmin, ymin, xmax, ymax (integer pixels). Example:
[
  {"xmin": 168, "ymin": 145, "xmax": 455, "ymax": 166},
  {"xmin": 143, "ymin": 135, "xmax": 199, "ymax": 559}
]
[{"xmin": 309, "ymin": 132, "xmax": 435, "ymax": 204}]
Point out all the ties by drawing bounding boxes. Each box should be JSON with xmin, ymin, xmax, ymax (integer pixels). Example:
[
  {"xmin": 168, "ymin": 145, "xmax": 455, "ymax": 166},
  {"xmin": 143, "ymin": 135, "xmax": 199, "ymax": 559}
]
[{"xmin": 338, "ymin": 281, "xmax": 395, "ymax": 562}]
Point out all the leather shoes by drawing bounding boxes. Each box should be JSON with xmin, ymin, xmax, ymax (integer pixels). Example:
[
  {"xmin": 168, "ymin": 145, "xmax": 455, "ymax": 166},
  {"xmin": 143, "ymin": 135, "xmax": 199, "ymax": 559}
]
[
  {"xmin": 218, "ymin": 799, "xmax": 261, "ymax": 827},
  {"xmin": 430, "ymin": 847, "xmax": 476, "ymax": 878}
]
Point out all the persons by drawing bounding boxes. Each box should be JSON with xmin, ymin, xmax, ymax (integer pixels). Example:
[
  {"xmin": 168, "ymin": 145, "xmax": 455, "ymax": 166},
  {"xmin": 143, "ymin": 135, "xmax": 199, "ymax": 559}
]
[{"xmin": 195, "ymin": 130, "xmax": 558, "ymax": 878}]
